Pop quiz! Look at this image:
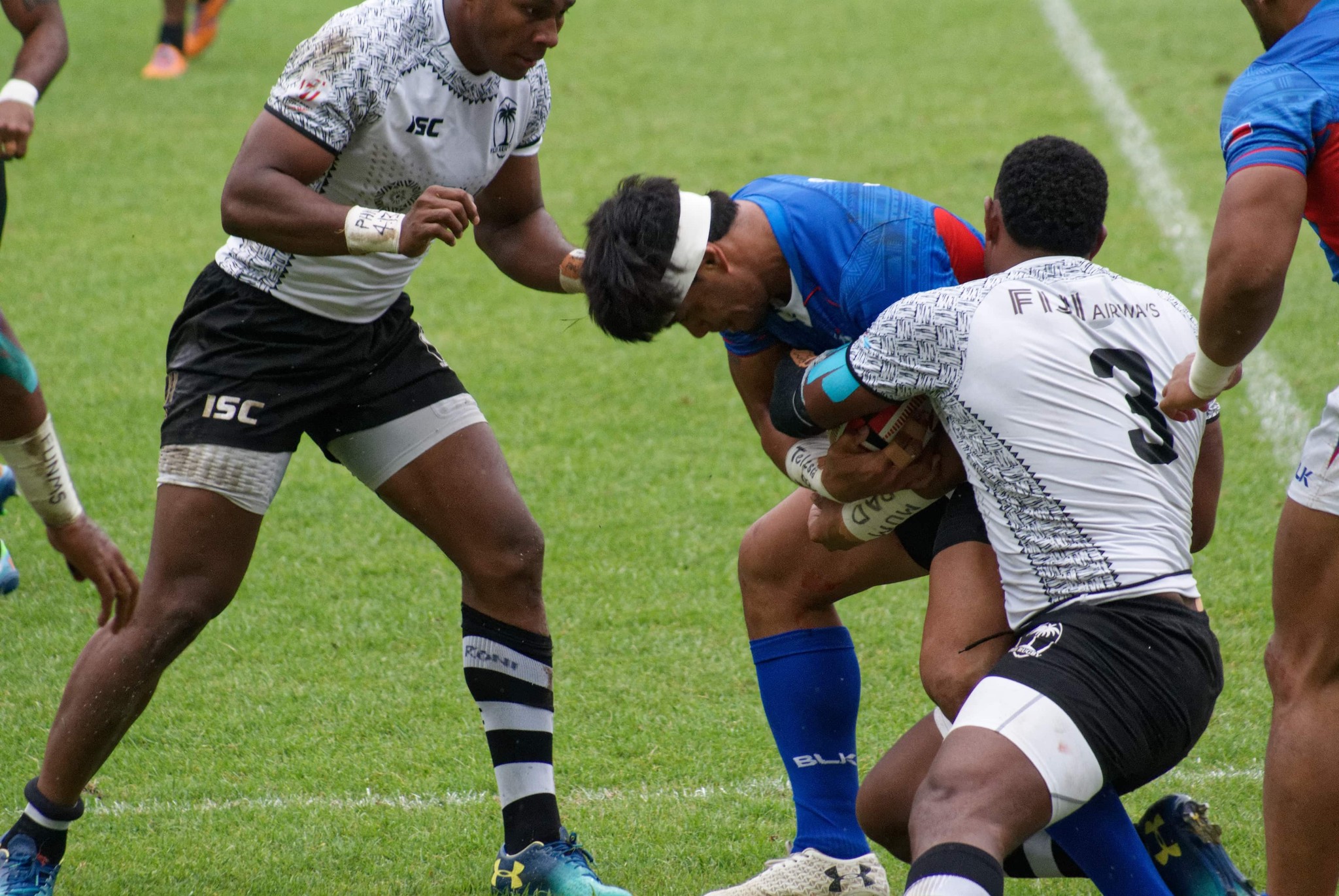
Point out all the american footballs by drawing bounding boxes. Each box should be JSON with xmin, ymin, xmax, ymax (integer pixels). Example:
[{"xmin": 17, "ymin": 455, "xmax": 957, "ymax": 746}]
[{"xmin": 826, "ymin": 393, "xmax": 935, "ymax": 472}]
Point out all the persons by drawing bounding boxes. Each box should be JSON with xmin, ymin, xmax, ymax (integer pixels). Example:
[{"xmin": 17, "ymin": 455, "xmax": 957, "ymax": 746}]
[
  {"xmin": 1160, "ymin": 0, "xmax": 1339, "ymax": 896},
  {"xmin": 581, "ymin": 173, "xmax": 1260, "ymax": 896},
  {"xmin": 0, "ymin": 0, "xmax": 141, "ymax": 635},
  {"xmin": 139, "ymin": 0, "xmax": 229, "ymax": 80},
  {"xmin": 769, "ymin": 136, "xmax": 1223, "ymax": 896},
  {"xmin": 0, "ymin": 0, "xmax": 590, "ymax": 896}
]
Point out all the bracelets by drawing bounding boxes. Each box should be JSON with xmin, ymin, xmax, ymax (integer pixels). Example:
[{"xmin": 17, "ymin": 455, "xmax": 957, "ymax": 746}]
[
  {"xmin": 841, "ymin": 489, "xmax": 945, "ymax": 542},
  {"xmin": 784, "ymin": 437, "xmax": 848, "ymax": 506},
  {"xmin": 0, "ymin": 78, "xmax": 40, "ymax": 106},
  {"xmin": 558, "ymin": 247, "xmax": 586, "ymax": 295},
  {"xmin": 1188, "ymin": 340, "xmax": 1243, "ymax": 400},
  {"xmin": 344, "ymin": 204, "xmax": 406, "ymax": 255}
]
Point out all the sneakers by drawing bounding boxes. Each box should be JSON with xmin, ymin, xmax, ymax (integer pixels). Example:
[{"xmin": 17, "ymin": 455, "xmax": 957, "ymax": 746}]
[
  {"xmin": 0, "ymin": 537, "xmax": 19, "ymax": 595},
  {"xmin": 182, "ymin": 0, "xmax": 229, "ymax": 57},
  {"xmin": 703, "ymin": 841, "xmax": 890, "ymax": 896},
  {"xmin": 1138, "ymin": 794, "xmax": 1264, "ymax": 896},
  {"xmin": 1, "ymin": 828, "xmax": 65, "ymax": 896},
  {"xmin": 141, "ymin": 43, "xmax": 188, "ymax": 78},
  {"xmin": 489, "ymin": 827, "xmax": 633, "ymax": 896},
  {"xmin": 1, "ymin": 465, "xmax": 19, "ymax": 519}
]
